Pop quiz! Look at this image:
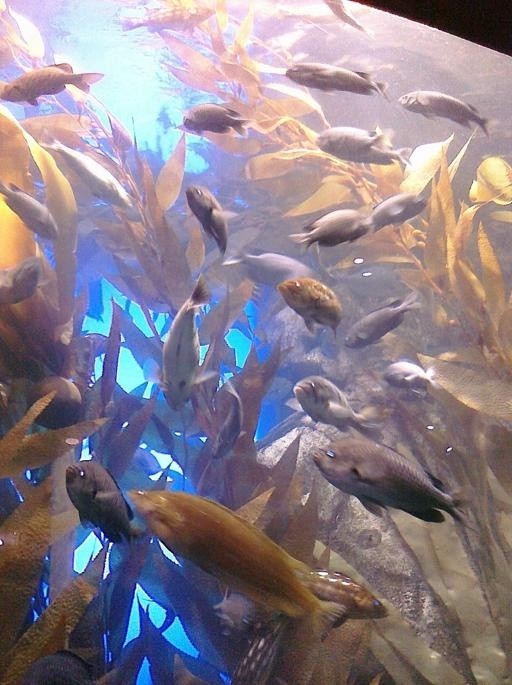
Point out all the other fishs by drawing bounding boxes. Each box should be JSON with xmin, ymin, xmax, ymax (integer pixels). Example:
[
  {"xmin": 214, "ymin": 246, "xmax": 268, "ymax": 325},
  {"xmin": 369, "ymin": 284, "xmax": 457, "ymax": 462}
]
[{"xmin": 143, "ymin": 184, "xmax": 440, "ymax": 461}]
